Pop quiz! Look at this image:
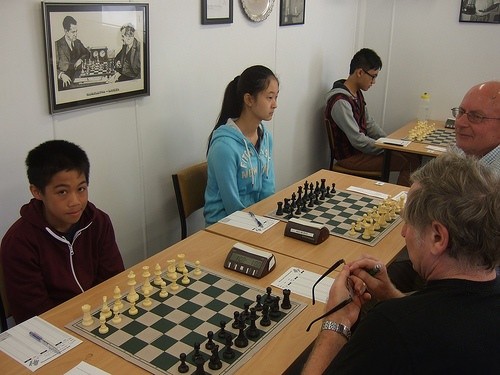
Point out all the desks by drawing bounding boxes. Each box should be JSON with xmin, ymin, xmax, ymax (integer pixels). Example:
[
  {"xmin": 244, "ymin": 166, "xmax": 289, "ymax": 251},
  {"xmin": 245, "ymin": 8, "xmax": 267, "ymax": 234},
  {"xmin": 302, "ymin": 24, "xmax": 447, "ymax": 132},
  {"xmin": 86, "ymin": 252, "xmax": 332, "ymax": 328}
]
[
  {"xmin": 375, "ymin": 119, "xmax": 456, "ymax": 184},
  {"xmin": 204, "ymin": 169, "xmax": 411, "ymax": 272},
  {"xmin": 0, "ymin": 230, "xmax": 339, "ymax": 375}
]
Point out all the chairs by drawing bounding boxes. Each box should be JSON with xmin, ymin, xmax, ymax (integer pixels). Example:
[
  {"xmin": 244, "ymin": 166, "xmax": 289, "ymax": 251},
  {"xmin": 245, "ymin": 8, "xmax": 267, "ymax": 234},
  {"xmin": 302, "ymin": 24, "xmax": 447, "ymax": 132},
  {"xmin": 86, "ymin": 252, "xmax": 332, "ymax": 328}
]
[
  {"xmin": 172, "ymin": 161, "xmax": 209, "ymax": 240},
  {"xmin": 323, "ymin": 109, "xmax": 383, "ymax": 180}
]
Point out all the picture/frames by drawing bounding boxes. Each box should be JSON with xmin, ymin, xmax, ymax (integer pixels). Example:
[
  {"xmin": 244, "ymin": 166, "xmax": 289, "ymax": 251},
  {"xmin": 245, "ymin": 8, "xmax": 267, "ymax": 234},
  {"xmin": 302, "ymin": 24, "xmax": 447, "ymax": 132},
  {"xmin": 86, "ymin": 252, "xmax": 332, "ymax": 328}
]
[
  {"xmin": 41, "ymin": 1, "xmax": 150, "ymax": 114},
  {"xmin": 458, "ymin": 0, "xmax": 500, "ymax": 23},
  {"xmin": 201, "ymin": 0, "xmax": 234, "ymax": 25},
  {"xmin": 280, "ymin": 0, "xmax": 305, "ymax": 26}
]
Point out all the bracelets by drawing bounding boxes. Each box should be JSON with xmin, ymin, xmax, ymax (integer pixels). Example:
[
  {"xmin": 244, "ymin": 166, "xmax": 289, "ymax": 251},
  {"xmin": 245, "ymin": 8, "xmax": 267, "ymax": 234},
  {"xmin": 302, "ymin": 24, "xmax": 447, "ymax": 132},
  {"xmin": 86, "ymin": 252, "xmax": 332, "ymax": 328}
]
[{"xmin": 321, "ymin": 320, "xmax": 351, "ymax": 341}]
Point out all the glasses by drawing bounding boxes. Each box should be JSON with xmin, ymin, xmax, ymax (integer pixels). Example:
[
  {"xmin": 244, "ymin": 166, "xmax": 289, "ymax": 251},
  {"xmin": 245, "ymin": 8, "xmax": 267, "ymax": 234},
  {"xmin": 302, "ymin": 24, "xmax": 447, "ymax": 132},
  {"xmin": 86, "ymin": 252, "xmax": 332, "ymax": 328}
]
[
  {"xmin": 357, "ymin": 67, "xmax": 377, "ymax": 80},
  {"xmin": 450, "ymin": 107, "xmax": 500, "ymax": 124}
]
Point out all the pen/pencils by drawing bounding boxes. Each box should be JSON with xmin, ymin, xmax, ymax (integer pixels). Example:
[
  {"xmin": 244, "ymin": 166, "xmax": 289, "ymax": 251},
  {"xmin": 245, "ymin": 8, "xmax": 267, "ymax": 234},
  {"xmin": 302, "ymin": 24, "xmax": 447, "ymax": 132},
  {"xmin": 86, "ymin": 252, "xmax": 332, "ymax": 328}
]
[
  {"xmin": 369, "ymin": 265, "xmax": 380, "ymax": 276},
  {"xmin": 249, "ymin": 211, "xmax": 263, "ymax": 228},
  {"xmin": 384, "ymin": 142, "xmax": 403, "ymax": 146},
  {"xmin": 29, "ymin": 331, "xmax": 60, "ymax": 353}
]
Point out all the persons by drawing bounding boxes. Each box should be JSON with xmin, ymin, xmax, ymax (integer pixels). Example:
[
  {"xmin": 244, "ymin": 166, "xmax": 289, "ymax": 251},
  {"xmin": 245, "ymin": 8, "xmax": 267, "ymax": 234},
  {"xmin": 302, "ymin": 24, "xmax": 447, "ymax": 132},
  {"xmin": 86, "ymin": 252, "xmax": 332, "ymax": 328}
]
[
  {"xmin": 455, "ymin": 81, "xmax": 500, "ymax": 178},
  {"xmin": 0, "ymin": 139, "xmax": 125, "ymax": 325},
  {"xmin": 301, "ymin": 149, "xmax": 500, "ymax": 375},
  {"xmin": 203, "ymin": 64, "xmax": 279, "ymax": 227},
  {"xmin": 326, "ymin": 48, "xmax": 419, "ymax": 187}
]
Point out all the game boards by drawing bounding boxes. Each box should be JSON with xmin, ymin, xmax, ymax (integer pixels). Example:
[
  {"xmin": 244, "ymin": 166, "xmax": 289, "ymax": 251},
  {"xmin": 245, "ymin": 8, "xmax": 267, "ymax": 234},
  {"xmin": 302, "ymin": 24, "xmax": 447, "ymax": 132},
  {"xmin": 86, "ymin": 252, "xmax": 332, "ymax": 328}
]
[
  {"xmin": 261, "ymin": 183, "xmax": 408, "ymax": 246},
  {"xmin": 399, "ymin": 124, "xmax": 458, "ymax": 148},
  {"xmin": 64, "ymin": 258, "xmax": 308, "ymax": 375}
]
